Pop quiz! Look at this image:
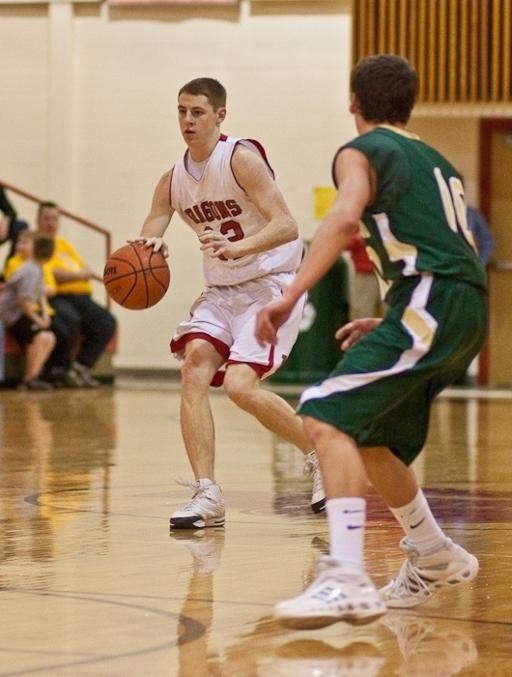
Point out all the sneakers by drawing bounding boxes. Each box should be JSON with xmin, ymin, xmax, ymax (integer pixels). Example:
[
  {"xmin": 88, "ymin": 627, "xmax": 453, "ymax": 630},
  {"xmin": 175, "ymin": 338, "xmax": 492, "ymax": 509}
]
[
  {"xmin": 279, "ymin": 641, "xmax": 382, "ymax": 672},
  {"xmin": 170, "ymin": 477, "xmax": 226, "ymax": 529},
  {"xmin": 377, "ymin": 537, "xmax": 479, "ymax": 610},
  {"xmin": 302, "ymin": 448, "xmax": 327, "ymax": 513},
  {"xmin": 272, "ymin": 555, "xmax": 387, "ymax": 629},
  {"xmin": 380, "ymin": 615, "xmax": 479, "ymax": 671},
  {"xmin": 16, "ymin": 363, "xmax": 99, "ymax": 392}
]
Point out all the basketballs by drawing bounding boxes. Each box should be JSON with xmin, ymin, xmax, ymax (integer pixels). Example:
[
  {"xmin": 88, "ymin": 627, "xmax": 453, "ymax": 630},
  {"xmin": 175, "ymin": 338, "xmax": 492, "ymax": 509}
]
[{"xmin": 104, "ymin": 244, "xmax": 170, "ymax": 308}]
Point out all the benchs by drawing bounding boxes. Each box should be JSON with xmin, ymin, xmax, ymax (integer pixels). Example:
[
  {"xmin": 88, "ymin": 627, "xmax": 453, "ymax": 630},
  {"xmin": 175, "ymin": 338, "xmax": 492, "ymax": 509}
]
[{"xmin": 0, "ymin": 305, "xmax": 117, "ymax": 386}]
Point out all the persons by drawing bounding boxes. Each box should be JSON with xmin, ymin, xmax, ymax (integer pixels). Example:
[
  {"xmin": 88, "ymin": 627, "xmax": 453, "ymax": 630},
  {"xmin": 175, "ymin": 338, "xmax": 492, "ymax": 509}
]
[
  {"xmin": 0, "ymin": 182, "xmax": 118, "ymax": 391},
  {"xmin": 454, "ymin": 206, "xmax": 496, "ymax": 388},
  {"xmin": 253, "ymin": 52, "xmax": 491, "ymax": 630},
  {"xmin": 124, "ymin": 77, "xmax": 328, "ymax": 530}
]
